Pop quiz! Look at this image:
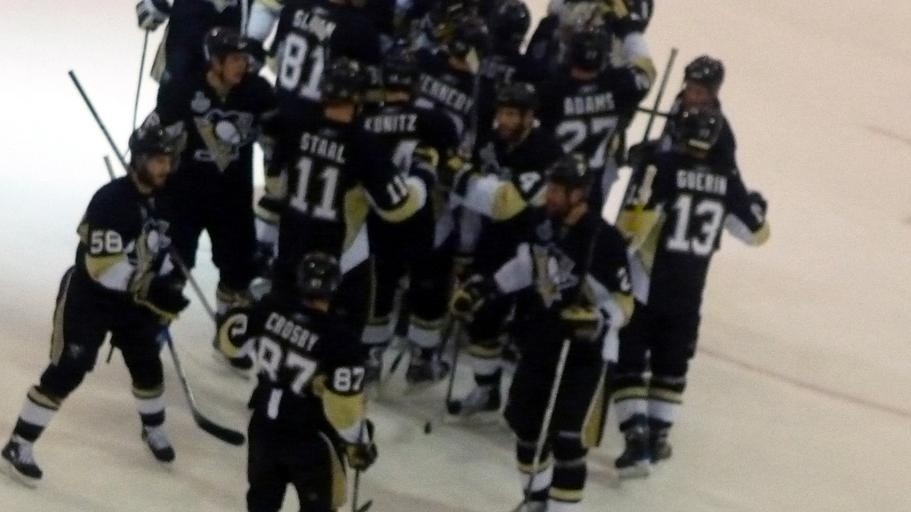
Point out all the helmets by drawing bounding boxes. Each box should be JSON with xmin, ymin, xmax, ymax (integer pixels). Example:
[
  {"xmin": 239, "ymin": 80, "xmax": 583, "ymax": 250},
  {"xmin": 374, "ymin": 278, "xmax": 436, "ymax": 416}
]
[
  {"xmin": 128, "ymin": 125, "xmax": 175, "ymax": 154},
  {"xmin": 297, "ymin": 253, "xmax": 345, "ymax": 293}
]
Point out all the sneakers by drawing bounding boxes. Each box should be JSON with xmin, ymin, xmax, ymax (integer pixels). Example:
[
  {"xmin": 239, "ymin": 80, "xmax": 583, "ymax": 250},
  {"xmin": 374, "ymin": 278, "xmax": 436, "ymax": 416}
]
[
  {"xmin": 141, "ymin": 425, "xmax": 175, "ymax": 462},
  {"xmin": 1, "ymin": 435, "xmax": 42, "ymax": 481},
  {"xmin": 616, "ymin": 439, "xmax": 672, "ymax": 469},
  {"xmin": 406, "ymin": 362, "xmax": 450, "ymax": 385},
  {"xmin": 446, "ymin": 386, "xmax": 500, "ymax": 416}
]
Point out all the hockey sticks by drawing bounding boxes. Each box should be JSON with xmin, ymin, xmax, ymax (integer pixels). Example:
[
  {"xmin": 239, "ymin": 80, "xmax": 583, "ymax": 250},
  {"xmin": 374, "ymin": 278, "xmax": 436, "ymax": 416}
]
[
  {"xmin": 100, "ymin": 156, "xmax": 250, "ymax": 448},
  {"xmin": 442, "ymin": 335, "xmax": 465, "ymax": 416},
  {"xmin": 352, "ymin": 467, "xmax": 373, "ymax": 511}
]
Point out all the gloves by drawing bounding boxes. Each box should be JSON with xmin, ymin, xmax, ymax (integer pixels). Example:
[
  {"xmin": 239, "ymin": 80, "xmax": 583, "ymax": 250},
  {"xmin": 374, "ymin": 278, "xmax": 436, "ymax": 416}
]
[
  {"xmin": 347, "ymin": 442, "xmax": 378, "ymax": 471},
  {"xmin": 132, "ymin": 271, "xmax": 189, "ymax": 318},
  {"xmin": 137, "ymin": 0, "xmax": 173, "ymax": 33}
]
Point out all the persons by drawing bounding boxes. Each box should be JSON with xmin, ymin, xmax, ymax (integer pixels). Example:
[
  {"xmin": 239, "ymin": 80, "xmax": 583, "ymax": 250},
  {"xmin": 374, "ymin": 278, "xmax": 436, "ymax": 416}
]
[
  {"xmin": 1, "ymin": 122, "xmax": 191, "ymax": 484},
  {"xmin": 126, "ymin": 0, "xmax": 772, "ymax": 511}
]
